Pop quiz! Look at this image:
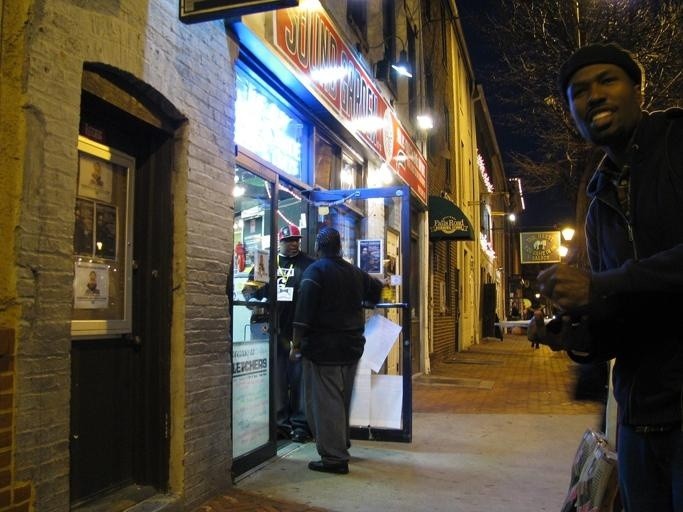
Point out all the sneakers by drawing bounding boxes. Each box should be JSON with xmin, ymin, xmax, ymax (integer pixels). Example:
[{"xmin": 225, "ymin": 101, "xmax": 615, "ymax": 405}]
[{"xmin": 275, "ymin": 431, "xmax": 307, "ymax": 442}]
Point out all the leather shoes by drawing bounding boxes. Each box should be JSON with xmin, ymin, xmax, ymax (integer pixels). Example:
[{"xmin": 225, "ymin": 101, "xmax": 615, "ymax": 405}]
[{"xmin": 308, "ymin": 459, "xmax": 350, "ymax": 474}]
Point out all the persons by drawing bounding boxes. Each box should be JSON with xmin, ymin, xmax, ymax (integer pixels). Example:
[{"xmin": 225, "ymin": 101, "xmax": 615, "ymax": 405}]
[
  {"xmin": 532, "ymin": 306, "xmax": 544, "ymax": 349},
  {"xmin": 288, "ymin": 226, "xmax": 383, "ymax": 474},
  {"xmin": 240, "ymin": 226, "xmax": 315, "ymax": 443},
  {"xmin": 526, "ymin": 43, "xmax": 683, "ymax": 512},
  {"xmin": 84, "ymin": 271, "xmax": 100, "ymax": 296},
  {"xmin": 511, "ymin": 301, "xmax": 519, "ymax": 316}
]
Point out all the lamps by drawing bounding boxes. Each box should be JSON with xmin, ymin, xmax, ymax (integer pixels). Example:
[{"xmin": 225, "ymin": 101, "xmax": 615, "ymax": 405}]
[{"xmin": 366, "ymin": 34, "xmax": 416, "ymax": 80}]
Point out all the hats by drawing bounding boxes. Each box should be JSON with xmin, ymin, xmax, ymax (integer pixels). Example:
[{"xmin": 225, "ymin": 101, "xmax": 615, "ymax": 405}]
[
  {"xmin": 279, "ymin": 224, "xmax": 302, "ymax": 239},
  {"xmin": 558, "ymin": 45, "xmax": 642, "ymax": 93}
]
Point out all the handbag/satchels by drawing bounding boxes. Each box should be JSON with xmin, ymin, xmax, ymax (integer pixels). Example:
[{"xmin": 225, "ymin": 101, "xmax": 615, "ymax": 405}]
[{"xmin": 560, "ymin": 421, "xmax": 623, "ymax": 512}]
